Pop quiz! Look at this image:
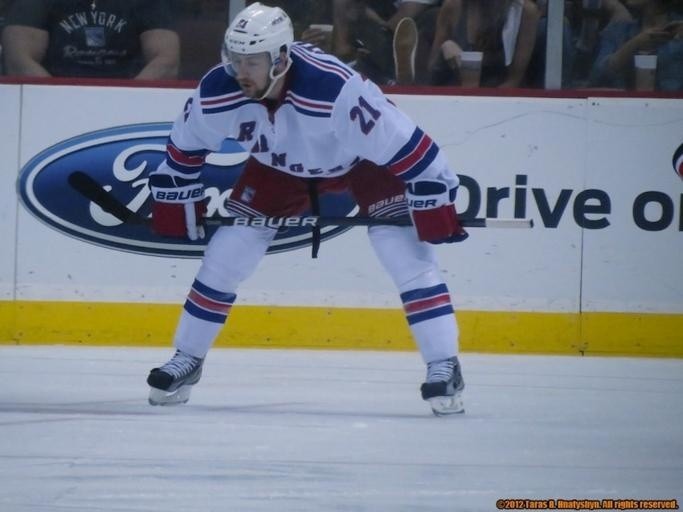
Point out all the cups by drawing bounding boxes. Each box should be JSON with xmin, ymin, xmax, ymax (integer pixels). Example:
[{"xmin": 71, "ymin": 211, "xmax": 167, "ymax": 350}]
[
  {"xmin": 634, "ymin": 53, "xmax": 658, "ymax": 92},
  {"xmin": 458, "ymin": 50, "xmax": 484, "ymax": 89}
]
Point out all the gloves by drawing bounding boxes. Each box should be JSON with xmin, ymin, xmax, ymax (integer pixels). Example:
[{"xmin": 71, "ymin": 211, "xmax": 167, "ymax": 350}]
[
  {"xmin": 405, "ymin": 178, "xmax": 469, "ymax": 245},
  {"xmin": 147, "ymin": 170, "xmax": 211, "ymax": 242}
]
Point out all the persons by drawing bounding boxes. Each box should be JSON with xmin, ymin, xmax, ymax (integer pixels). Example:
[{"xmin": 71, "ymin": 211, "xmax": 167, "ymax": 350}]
[
  {"xmin": 0, "ymin": 0, "xmax": 183, "ymax": 83},
  {"xmin": 135, "ymin": 2, "xmax": 471, "ymax": 401},
  {"xmin": 284, "ymin": 0, "xmax": 681, "ymax": 90}
]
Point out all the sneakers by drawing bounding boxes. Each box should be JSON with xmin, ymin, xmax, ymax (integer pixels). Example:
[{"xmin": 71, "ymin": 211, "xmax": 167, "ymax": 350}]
[
  {"xmin": 393, "ymin": 17, "xmax": 419, "ymax": 86},
  {"xmin": 147, "ymin": 348, "xmax": 205, "ymax": 393},
  {"xmin": 421, "ymin": 356, "xmax": 465, "ymax": 401}
]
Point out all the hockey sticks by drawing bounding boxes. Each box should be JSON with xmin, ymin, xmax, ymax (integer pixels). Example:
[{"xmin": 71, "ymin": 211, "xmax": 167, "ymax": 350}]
[{"xmin": 69, "ymin": 171, "xmax": 534, "ymax": 229}]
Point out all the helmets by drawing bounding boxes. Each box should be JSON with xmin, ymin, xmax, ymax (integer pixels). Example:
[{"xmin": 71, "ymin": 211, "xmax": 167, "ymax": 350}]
[{"xmin": 224, "ymin": 1, "xmax": 294, "ymax": 67}]
